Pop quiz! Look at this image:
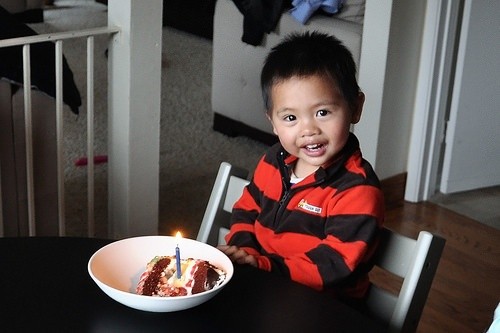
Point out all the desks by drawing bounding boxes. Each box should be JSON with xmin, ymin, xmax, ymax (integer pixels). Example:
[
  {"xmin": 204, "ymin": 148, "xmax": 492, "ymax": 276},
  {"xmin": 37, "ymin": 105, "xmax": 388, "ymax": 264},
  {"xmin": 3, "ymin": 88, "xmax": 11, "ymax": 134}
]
[{"xmin": 0, "ymin": 237, "xmax": 386, "ymax": 333}]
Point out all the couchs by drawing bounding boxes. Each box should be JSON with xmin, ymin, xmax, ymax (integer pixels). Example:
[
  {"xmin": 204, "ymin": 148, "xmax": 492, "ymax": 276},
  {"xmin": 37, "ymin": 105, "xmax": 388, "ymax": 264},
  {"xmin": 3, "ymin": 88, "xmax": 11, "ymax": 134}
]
[{"xmin": 210, "ymin": 0, "xmax": 365, "ymax": 146}]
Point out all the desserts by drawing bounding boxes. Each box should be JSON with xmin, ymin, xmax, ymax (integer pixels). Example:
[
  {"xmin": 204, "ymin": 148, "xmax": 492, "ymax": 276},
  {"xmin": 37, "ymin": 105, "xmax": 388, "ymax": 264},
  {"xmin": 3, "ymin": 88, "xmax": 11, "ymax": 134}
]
[{"xmin": 135, "ymin": 255, "xmax": 225, "ymax": 297}]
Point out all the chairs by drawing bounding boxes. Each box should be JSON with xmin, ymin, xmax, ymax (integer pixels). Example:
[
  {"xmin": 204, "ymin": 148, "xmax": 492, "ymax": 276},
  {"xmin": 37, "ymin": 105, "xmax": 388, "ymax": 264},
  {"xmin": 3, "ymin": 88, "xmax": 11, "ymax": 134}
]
[{"xmin": 197, "ymin": 161, "xmax": 446, "ymax": 333}]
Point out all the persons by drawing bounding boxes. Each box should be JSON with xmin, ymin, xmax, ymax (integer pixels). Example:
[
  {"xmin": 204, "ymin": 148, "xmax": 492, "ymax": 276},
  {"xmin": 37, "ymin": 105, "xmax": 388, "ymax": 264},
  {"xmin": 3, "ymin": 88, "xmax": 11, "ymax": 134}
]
[{"xmin": 216, "ymin": 30, "xmax": 386, "ymax": 303}]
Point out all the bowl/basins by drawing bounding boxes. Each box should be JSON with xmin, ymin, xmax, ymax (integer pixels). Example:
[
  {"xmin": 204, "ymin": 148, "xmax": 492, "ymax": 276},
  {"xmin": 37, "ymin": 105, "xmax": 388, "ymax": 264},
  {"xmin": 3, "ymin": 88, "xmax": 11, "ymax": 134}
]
[{"xmin": 88, "ymin": 235, "xmax": 234, "ymax": 312}]
